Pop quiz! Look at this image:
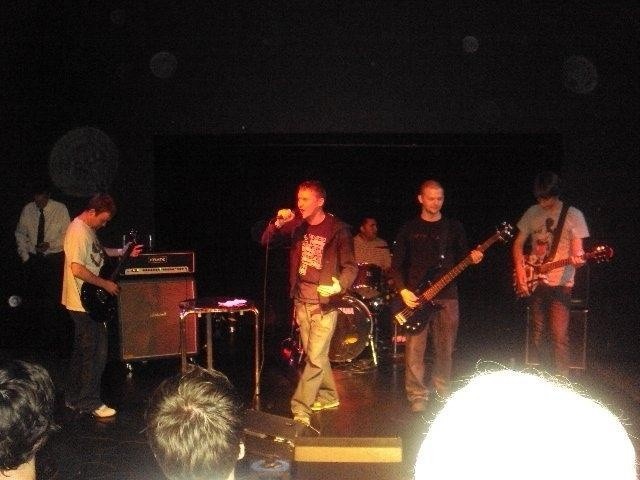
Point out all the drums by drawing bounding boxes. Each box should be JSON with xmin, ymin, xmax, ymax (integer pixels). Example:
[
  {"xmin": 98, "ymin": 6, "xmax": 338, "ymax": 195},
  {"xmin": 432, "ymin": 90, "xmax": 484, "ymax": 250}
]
[
  {"xmin": 329, "ymin": 295, "xmax": 373, "ymax": 362},
  {"xmin": 351, "ymin": 262, "xmax": 387, "ymax": 298}
]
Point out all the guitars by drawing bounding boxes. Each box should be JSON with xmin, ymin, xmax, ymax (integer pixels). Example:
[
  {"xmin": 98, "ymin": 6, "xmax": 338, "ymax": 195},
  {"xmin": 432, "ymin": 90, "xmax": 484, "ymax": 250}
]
[
  {"xmin": 392, "ymin": 222, "xmax": 515, "ymax": 336},
  {"xmin": 81, "ymin": 230, "xmax": 143, "ymax": 323},
  {"xmin": 512, "ymin": 244, "xmax": 614, "ymax": 297}
]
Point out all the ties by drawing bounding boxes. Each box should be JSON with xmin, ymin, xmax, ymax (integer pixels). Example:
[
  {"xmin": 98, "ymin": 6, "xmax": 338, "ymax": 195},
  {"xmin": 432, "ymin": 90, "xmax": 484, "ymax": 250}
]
[{"xmin": 36, "ymin": 208, "xmax": 45, "ymax": 247}]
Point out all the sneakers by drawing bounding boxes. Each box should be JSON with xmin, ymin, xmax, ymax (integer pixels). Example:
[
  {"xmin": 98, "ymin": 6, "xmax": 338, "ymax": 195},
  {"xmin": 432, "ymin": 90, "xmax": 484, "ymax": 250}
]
[
  {"xmin": 291, "ymin": 416, "xmax": 310, "ymax": 429},
  {"xmin": 311, "ymin": 398, "xmax": 341, "ymax": 412},
  {"xmin": 412, "ymin": 397, "xmax": 426, "ymax": 413},
  {"xmin": 91, "ymin": 401, "xmax": 116, "ymax": 420}
]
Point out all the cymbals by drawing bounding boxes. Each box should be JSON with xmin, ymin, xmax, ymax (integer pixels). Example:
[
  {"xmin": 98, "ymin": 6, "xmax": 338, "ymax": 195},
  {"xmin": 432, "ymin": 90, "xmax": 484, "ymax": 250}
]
[{"xmin": 375, "ymin": 243, "xmax": 404, "ymax": 251}]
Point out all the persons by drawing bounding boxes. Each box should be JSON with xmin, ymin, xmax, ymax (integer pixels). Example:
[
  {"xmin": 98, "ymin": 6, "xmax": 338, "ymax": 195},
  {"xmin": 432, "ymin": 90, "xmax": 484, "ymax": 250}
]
[
  {"xmin": 512, "ymin": 171, "xmax": 588, "ymax": 378},
  {"xmin": 352, "ymin": 218, "xmax": 392, "ymax": 271},
  {"xmin": 60, "ymin": 192, "xmax": 143, "ymax": 416},
  {"xmin": 260, "ymin": 180, "xmax": 359, "ymax": 427},
  {"xmin": 15, "ymin": 182, "xmax": 71, "ymax": 298},
  {"xmin": 0, "ymin": 358, "xmax": 61, "ymax": 480},
  {"xmin": 145, "ymin": 364, "xmax": 245, "ymax": 480},
  {"xmin": 391, "ymin": 180, "xmax": 484, "ymax": 413}
]
